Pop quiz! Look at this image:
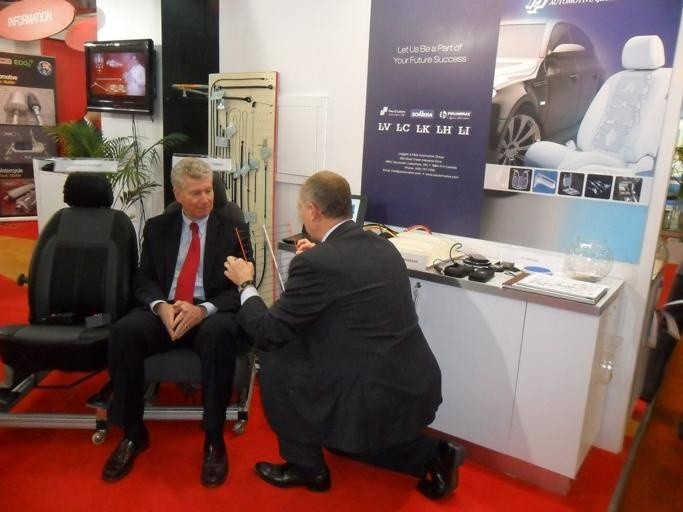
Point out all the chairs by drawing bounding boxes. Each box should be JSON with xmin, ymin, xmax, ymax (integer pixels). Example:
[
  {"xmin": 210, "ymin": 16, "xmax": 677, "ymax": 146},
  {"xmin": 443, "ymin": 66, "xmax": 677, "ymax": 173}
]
[
  {"xmin": 1, "ymin": 172, "xmax": 138, "ymax": 445},
  {"xmin": 140, "ymin": 169, "xmax": 262, "ymax": 435}
]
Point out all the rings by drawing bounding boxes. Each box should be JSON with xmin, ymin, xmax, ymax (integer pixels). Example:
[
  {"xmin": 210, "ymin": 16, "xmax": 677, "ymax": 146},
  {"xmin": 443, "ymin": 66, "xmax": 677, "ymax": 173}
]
[{"xmin": 184, "ymin": 324, "xmax": 189, "ymax": 329}]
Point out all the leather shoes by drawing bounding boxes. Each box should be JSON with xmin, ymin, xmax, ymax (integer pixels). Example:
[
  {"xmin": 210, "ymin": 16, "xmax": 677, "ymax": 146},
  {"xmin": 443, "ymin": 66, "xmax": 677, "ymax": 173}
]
[
  {"xmin": 416, "ymin": 438, "xmax": 466, "ymax": 500},
  {"xmin": 200, "ymin": 440, "xmax": 229, "ymax": 487},
  {"xmin": 254, "ymin": 460, "xmax": 332, "ymax": 492},
  {"xmin": 102, "ymin": 434, "xmax": 150, "ymax": 482}
]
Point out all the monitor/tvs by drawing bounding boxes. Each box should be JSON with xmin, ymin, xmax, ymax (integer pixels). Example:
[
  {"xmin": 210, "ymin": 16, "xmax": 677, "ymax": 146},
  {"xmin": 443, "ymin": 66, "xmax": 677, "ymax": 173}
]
[{"xmin": 84, "ymin": 39, "xmax": 154, "ymax": 113}]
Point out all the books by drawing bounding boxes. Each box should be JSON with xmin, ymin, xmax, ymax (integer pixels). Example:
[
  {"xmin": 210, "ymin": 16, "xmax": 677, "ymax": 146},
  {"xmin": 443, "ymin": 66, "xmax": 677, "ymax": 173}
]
[{"xmin": 502, "ymin": 270, "xmax": 608, "ymax": 305}]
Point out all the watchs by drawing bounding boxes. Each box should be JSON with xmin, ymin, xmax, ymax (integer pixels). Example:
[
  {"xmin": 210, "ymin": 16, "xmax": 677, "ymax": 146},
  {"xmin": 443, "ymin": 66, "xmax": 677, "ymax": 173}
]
[{"xmin": 237, "ymin": 281, "xmax": 255, "ymax": 294}]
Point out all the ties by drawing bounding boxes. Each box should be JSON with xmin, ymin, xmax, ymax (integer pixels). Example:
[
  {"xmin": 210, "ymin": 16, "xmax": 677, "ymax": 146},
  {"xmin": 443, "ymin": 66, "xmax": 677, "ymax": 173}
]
[{"xmin": 173, "ymin": 222, "xmax": 202, "ymax": 306}]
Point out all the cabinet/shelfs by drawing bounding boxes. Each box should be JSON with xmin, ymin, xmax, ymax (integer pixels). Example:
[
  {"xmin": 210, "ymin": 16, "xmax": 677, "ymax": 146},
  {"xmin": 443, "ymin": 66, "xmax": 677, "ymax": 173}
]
[{"xmin": 277, "ymin": 225, "xmax": 626, "ymax": 499}]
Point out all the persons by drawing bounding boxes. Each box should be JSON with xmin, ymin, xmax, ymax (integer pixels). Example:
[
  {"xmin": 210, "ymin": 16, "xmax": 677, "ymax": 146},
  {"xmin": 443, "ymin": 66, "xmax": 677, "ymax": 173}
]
[
  {"xmin": 224, "ymin": 169, "xmax": 465, "ymax": 499},
  {"xmin": 123, "ymin": 55, "xmax": 146, "ymax": 95},
  {"xmin": 102, "ymin": 156, "xmax": 254, "ymax": 487},
  {"xmin": 74, "ymin": 105, "xmax": 102, "ymax": 157}
]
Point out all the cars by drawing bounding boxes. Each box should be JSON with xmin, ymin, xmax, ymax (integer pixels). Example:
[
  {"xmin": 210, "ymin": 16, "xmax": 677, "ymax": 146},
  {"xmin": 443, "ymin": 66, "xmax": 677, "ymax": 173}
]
[{"xmin": 485, "ymin": 16, "xmax": 604, "ymax": 169}]
